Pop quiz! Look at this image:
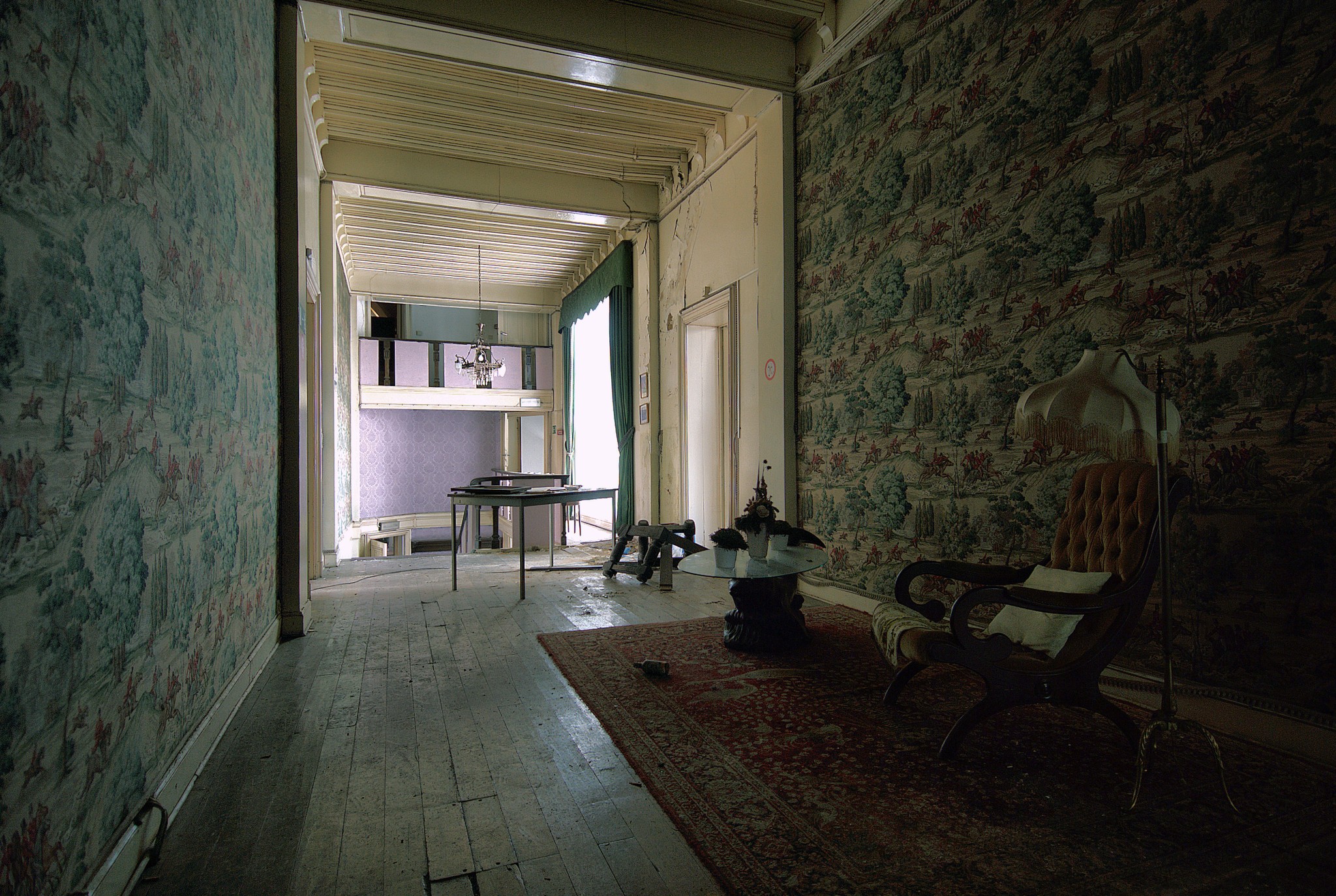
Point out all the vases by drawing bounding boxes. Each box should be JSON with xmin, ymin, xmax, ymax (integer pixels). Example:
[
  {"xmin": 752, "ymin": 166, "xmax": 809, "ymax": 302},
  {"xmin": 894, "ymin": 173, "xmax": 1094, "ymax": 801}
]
[{"xmin": 747, "ymin": 528, "xmax": 769, "ymax": 557}]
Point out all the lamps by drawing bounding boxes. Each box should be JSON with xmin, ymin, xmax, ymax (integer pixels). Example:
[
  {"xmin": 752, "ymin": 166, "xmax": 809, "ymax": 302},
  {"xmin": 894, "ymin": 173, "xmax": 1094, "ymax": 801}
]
[
  {"xmin": 455, "ymin": 245, "xmax": 506, "ymax": 385},
  {"xmin": 1016, "ymin": 348, "xmax": 1237, "ymax": 813}
]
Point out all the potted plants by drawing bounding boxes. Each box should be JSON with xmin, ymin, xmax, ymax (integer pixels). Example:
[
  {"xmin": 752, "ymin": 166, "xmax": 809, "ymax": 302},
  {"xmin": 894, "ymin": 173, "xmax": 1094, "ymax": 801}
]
[
  {"xmin": 771, "ymin": 520, "xmax": 791, "ymax": 551},
  {"xmin": 709, "ymin": 528, "xmax": 749, "ymax": 568}
]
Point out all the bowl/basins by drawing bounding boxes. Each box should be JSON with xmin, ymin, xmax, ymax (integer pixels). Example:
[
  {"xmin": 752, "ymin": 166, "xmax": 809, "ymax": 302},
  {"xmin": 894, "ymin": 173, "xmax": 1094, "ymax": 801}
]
[{"xmin": 623, "ymin": 548, "xmax": 631, "ymax": 554}]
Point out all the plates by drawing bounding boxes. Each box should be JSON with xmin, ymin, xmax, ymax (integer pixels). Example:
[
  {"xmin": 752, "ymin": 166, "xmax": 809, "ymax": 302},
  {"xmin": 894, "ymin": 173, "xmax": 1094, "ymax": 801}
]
[{"xmin": 565, "ymin": 487, "xmax": 578, "ymax": 490}]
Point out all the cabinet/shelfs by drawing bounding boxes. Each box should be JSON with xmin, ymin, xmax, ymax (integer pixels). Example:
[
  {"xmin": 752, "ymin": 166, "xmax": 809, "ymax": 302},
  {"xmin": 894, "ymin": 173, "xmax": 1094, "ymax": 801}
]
[{"xmin": 357, "ymin": 298, "xmax": 552, "ymax": 348}]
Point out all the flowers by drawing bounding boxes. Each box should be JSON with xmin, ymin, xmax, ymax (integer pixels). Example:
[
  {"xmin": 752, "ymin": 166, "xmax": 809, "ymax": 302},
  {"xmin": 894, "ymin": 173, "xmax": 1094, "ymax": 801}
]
[{"xmin": 737, "ymin": 460, "xmax": 779, "ymax": 526}]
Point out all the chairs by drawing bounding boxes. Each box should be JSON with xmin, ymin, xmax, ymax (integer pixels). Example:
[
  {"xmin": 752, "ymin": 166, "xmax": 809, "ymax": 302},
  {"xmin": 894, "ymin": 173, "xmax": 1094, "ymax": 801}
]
[{"xmin": 871, "ymin": 462, "xmax": 1193, "ymax": 760}]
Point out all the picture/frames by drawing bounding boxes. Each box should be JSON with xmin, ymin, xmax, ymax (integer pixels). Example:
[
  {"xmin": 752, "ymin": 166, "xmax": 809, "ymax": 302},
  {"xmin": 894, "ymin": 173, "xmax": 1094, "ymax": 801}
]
[
  {"xmin": 639, "ymin": 372, "xmax": 648, "ymax": 398},
  {"xmin": 639, "ymin": 402, "xmax": 649, "ymax": 424}
]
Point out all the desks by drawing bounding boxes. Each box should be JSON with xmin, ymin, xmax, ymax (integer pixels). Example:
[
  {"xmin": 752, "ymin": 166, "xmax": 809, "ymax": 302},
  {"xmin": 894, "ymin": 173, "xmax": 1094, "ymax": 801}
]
[
  {"xmin": 677, "ymin": 546, "xmax": 828, "ymax": 648},
  {"xmin": 447, "ymin": 486, "xmax": 620, "ymax": 599}
]
